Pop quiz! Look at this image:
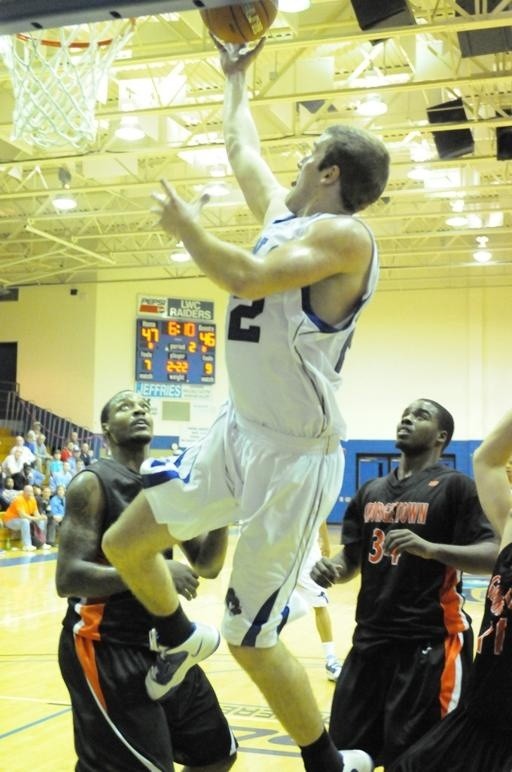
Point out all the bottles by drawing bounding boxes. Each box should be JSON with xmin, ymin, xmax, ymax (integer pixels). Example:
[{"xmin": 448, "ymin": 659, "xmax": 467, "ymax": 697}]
[{"xmin": 6, "ymin": 538, "xmax": 11, "ymax": 550}]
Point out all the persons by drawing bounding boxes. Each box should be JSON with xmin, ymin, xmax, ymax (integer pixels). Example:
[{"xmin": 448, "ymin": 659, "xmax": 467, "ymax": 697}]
[
  {"xmin": 102, "ymin": 27, "xmax": 388, "ymax": 772},
  {"xmin": 51, "ymin": 389, "xmax": 270, "ymax": 770},
  {"xmin": 309, "ymin": 394, "xmax": 502, "ymax": 770},
  {"xmin": 2, "ymin": 419, "xmax": 105, "ymax": 550},
  {"xmin": 276, "ymin": 514, "xmax": 346, "ymax": 686},
  {"xmin": 385, "ymin": 416, "xmax": 511, "ymax": 772}
]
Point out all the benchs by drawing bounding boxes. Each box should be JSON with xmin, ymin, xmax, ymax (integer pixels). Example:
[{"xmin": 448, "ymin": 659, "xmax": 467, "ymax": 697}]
[{"xmin": 0, "ymin": 427, "xmax": 70, "ymax": 550}]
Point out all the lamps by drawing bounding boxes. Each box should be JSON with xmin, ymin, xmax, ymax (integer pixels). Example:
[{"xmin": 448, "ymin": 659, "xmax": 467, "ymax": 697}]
[
  {"xmin": 357, "ymin": 60, "xmax": 387, "ymax": 116},
  {"xmin": 114, "ymin": 87, "xmax": 144, "ymax": 141},
  {"xmin": 170, "ymin": 240, "xmax": 192, "ymax": 263},
  {"xmin": 473, "ymin": 236, "xmax": 493, "ymax": 262},
  {"xmin": 52, "ymin": 167, "xmax": 76, "ymax": 210}
]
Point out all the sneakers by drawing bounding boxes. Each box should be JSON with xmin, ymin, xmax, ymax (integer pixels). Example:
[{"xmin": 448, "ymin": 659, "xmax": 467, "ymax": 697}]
[
  {"xmin": 338, "ymin": 748, "xmax": 375, "ymax": 772},
  {"xmin": 326, "ymin": 662, "xmax": 343, "ymax": 682},
  {"xmin": 144, "ymin": 622, "xmax": 221, "ymax": 704}
]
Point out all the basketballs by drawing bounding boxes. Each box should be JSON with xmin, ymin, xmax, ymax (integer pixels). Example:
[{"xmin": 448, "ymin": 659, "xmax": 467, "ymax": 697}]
[{"xmin": 199, "ymin": 0, "xmax": 276, "ymax": 44}]
